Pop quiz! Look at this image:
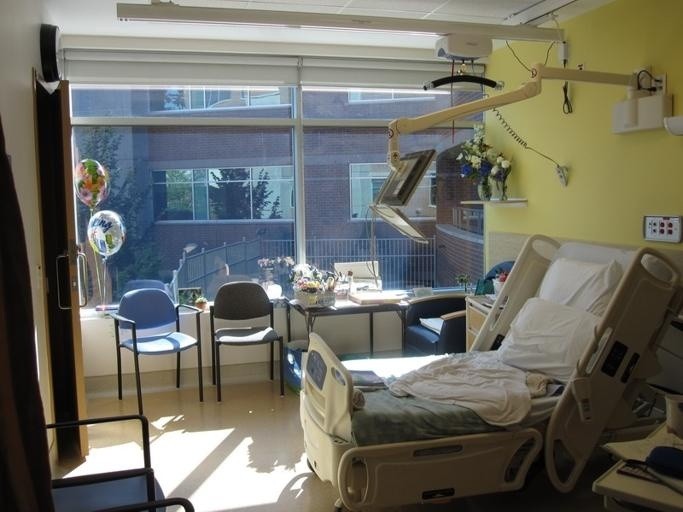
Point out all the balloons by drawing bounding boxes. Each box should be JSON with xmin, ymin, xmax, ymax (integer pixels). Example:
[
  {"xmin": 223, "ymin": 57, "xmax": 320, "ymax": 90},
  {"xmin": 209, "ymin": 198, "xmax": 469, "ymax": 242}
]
[
  {"xmin": 75, "ymin": 158, "xmax": 108, "ymax": 210},
  {"xmin": 87, "ymin": 209, "xmax": 125, "ymax": 257}
]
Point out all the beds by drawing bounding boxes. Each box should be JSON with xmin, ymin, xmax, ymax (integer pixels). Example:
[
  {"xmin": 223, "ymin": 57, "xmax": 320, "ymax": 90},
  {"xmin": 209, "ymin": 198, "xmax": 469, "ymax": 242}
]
[{"xmin": 294, "ymin": 233, "xmax": 679, "ymax": 512}]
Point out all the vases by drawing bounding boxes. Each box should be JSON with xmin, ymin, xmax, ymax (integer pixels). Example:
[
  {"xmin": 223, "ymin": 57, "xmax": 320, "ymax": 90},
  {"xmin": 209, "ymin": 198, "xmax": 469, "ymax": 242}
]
[
  {"xmin": 474, "ymin": 176, "xmax": 507, "ymax": 200},
  {"xmin": 492, "ymin": 278, "xmax": 504, "ymax": 300}
]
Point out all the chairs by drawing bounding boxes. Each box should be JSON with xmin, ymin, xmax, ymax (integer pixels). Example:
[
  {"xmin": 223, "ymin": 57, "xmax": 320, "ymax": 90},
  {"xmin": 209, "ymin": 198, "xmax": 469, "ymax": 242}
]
[
  {"xmin": 107, "ymin": 277, "xmax": 283, "ymax": 413},
  {"xmin": 39, "ymin": 410, "xmax": 195, "ymax": 509},
  {"xmin": 401, "ymin": 261, "xmax": 514, "ymax": 358}
]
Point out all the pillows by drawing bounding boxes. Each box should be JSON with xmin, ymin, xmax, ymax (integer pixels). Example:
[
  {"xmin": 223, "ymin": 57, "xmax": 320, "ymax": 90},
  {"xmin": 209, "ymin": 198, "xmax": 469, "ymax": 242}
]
[{"xmin": 495, "ymin": 298, "xmax": 603, "ymax": 384}]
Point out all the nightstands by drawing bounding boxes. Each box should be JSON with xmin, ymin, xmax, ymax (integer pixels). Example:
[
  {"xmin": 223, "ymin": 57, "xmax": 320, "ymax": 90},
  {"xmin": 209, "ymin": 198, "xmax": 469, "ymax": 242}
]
[
  {"xmin": 464, "ymin": 294, "xmax": 497, "ymax": 354},
  {"xmin": 283, "ymin": 294, "xmax": 410, "ymax": 364}
]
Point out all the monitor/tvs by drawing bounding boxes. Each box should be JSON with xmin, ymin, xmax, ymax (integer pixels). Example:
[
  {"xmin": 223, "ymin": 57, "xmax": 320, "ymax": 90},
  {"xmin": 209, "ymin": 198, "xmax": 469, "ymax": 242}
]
[{"xmin": 374, "ymin": 149, "xmax": 436, "ymax": 206}]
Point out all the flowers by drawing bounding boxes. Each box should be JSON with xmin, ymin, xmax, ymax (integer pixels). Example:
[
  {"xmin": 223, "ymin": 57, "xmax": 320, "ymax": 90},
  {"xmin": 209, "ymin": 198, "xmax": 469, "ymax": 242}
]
[
  {"xmin": 457, "ymin": 124, "xmax": 512, "ymax": 183},
  {"xmin": 287, "ymin": 261, "xmax": 337, "ymax": 296},
  {"xmin": 494, "ymin": 268, "xmax": 508, "ymax": 282},
  {"xmin": 256, "ymin": 255, "xmax": 294, "ymax": 268}
]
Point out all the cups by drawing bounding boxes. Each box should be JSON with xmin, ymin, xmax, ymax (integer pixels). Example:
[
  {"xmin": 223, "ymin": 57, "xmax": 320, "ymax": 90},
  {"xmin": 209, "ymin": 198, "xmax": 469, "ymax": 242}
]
[{"xmin": 663, "ymin": 391, "xmax": 683, "ymax": 436}]
